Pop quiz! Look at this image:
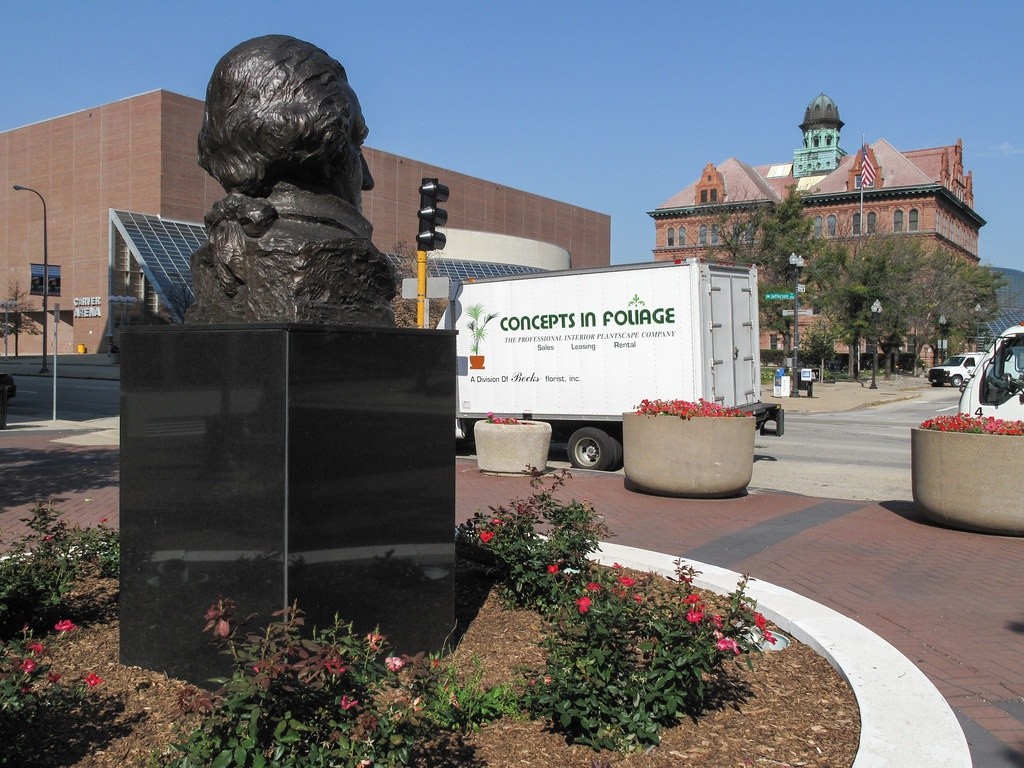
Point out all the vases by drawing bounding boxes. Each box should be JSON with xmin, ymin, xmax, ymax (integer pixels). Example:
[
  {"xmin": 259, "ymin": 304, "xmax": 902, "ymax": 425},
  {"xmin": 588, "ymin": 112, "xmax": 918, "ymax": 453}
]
[
  {"xmin": 474, "ymin": 420, "xmax": 552, "ymax": 477},
  {"xmin": 911, "ymin": 428, "xmax": 1024, "ymax": 537},
  {"xmin": 622, "ymin": 412, "xmax": 756, "ymax": 498}
]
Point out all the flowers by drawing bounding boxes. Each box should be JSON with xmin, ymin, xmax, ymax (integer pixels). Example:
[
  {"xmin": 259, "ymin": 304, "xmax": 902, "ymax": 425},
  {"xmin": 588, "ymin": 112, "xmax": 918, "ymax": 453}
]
[
  {"xmin": 632, "ymin": 397, "xmax": 752, "ymax": 422},
  {"xmin": 485, "ymin": 412, "xmax": 535, "ymax": 425},
  {"xmin": 918, "ymin": 413, "xmax": 1024, "ymax": 436}
]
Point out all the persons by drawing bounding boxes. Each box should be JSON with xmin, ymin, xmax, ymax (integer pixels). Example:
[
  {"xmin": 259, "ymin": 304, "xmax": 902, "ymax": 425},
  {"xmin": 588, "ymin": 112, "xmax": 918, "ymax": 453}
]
[{"xmin": 181, "ymin": 33, "xmax": 402, "ymax": 325}]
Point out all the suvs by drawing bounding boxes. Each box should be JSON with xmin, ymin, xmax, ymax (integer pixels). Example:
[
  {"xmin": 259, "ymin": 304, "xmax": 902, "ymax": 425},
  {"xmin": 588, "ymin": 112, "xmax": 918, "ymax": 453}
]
[{"xmin": 927, "ymin": 352, "xmax": 988, "ymax": 387}]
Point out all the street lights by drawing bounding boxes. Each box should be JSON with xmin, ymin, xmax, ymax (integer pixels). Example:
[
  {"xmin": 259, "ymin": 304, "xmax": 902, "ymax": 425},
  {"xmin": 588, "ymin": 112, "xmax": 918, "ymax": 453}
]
[
  {"xmin": 974, "ymin": 303, "xmax": 981, "ymax": 352},
  {"xmin": 12, "ymin": 182, "xmax": 48, "ymax": 374},
  {"xmin": 788, "ymin": 251, "xmax": 805, "ymax": 398},
  {"xmin": 869, "ymin": 298, "xmax": 882, "ymax": 389},
  {"xmin": 939, "ymin": 313, "xmax": 946, "ymax": 366}
]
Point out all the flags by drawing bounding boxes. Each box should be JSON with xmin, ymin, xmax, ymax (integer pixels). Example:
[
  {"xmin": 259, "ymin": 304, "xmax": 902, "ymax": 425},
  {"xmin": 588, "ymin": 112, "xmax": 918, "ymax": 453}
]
[{"xmin": 860, "ymin": 147, "xmax": 877, "ymax": 188}]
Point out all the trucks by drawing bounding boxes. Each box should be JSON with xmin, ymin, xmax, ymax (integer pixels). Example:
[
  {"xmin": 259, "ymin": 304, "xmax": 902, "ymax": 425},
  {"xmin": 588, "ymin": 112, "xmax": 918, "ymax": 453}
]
[
  {"xmin": 957, "ymin": 320, "xmax": 1024, "ymax": 426},
  {"xmin": 433, "ymin": 255, "xmax": 787, "ymax": 475}
]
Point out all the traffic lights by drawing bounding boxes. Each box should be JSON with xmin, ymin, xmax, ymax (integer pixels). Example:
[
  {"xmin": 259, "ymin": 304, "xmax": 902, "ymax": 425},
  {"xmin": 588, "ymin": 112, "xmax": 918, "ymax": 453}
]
[{"xmin": 414, "ymin": 176, "xmax": 450, "ymax": 251}]
